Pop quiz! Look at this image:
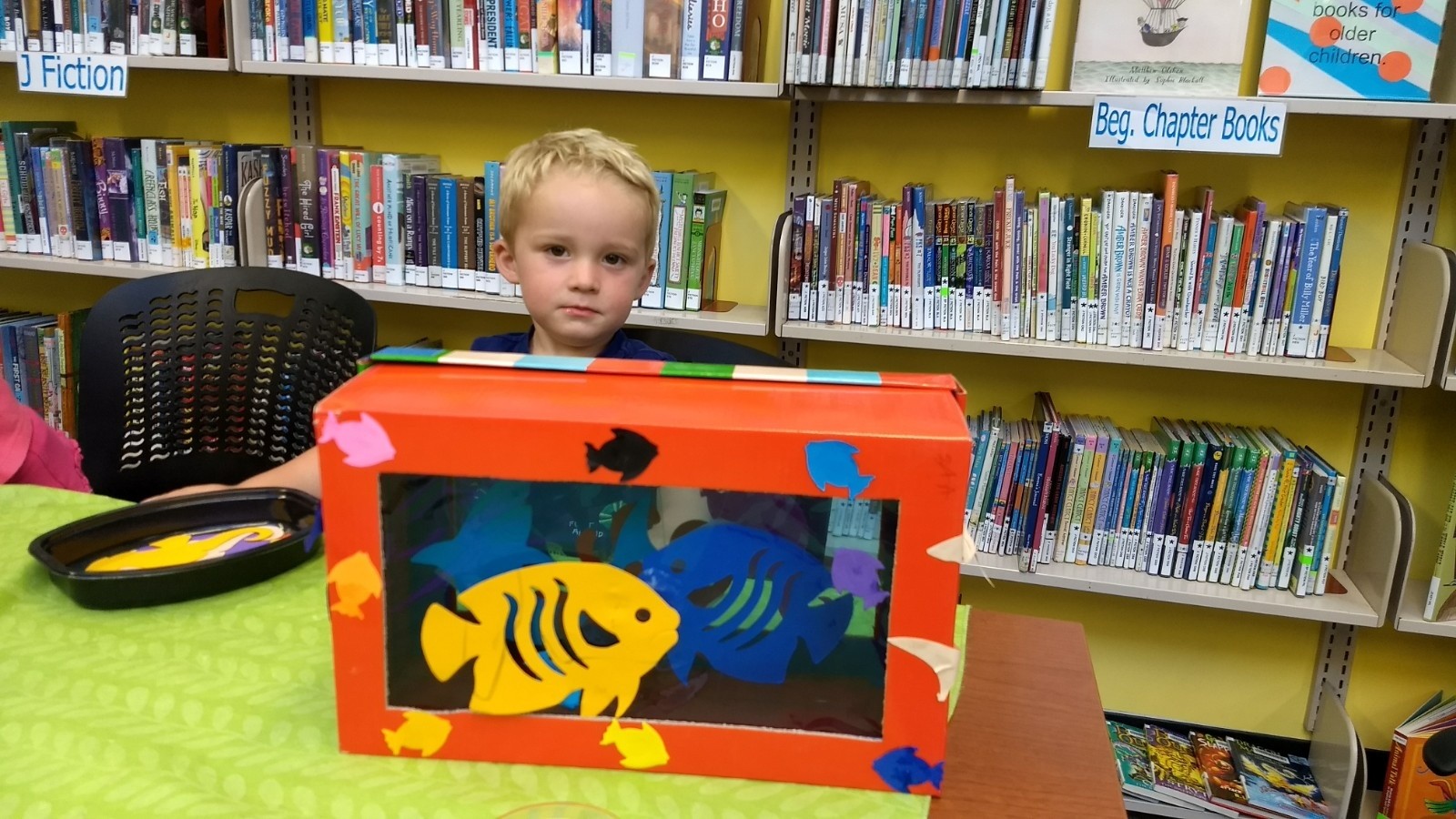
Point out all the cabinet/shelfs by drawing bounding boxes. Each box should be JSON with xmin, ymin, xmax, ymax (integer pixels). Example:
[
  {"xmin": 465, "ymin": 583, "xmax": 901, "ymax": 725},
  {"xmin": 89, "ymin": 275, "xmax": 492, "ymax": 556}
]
[{"xmin": 0, "ymin": 0, "xmax": 1456, "ymax": 819}]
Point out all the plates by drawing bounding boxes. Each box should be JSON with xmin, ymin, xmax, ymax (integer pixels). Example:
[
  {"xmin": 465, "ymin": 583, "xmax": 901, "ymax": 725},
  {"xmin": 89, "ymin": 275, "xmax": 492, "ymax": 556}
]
[{"xmin": 28, "ymin": 487, "xmax": 321, "ymax": 610}]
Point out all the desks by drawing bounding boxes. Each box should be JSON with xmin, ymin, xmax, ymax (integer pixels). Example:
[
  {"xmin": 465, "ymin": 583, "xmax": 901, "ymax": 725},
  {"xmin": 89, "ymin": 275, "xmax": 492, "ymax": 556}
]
[{"xmin": 0, "ymin": 484, "xmax": 1125, "ymax": 819}]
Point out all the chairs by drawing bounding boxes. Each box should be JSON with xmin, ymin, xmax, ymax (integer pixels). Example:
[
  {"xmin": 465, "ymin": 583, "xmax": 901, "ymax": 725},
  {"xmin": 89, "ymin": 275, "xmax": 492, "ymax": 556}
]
[{"xmin": 76, "ymin": 263, "xmax": 377, "ymax": 501}]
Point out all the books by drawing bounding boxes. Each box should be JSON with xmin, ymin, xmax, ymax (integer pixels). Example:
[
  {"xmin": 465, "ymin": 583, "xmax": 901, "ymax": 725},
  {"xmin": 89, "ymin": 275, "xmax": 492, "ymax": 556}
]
[{"xmin": 1, "ymin": 0, "xmax": 1456, "ymax": 819}]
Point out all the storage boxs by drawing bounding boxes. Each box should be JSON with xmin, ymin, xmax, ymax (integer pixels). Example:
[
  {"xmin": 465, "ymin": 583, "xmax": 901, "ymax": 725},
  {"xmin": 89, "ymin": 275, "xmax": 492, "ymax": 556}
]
[
  {"xmin": 1069, "ymin": 0, "xmax": 1263, "ymax": 96},
  {"xmin": 1257, "ymin": 0, "xmax": 1449, "ymax": 102},
  {"xmin": 314, "ymin": 345, "xmax": 970, "ymax": 795}
]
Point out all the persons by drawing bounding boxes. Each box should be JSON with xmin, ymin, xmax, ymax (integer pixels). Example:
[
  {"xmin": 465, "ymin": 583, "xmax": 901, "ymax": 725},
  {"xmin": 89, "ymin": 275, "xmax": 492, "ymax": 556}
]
[
  {"xmin": 143, "ymin": 127, "xmax": 671, "ymax": 504},
  {"xmin": 0, "ymin": 376, "xmax": 97, "ymax": 496}
]
